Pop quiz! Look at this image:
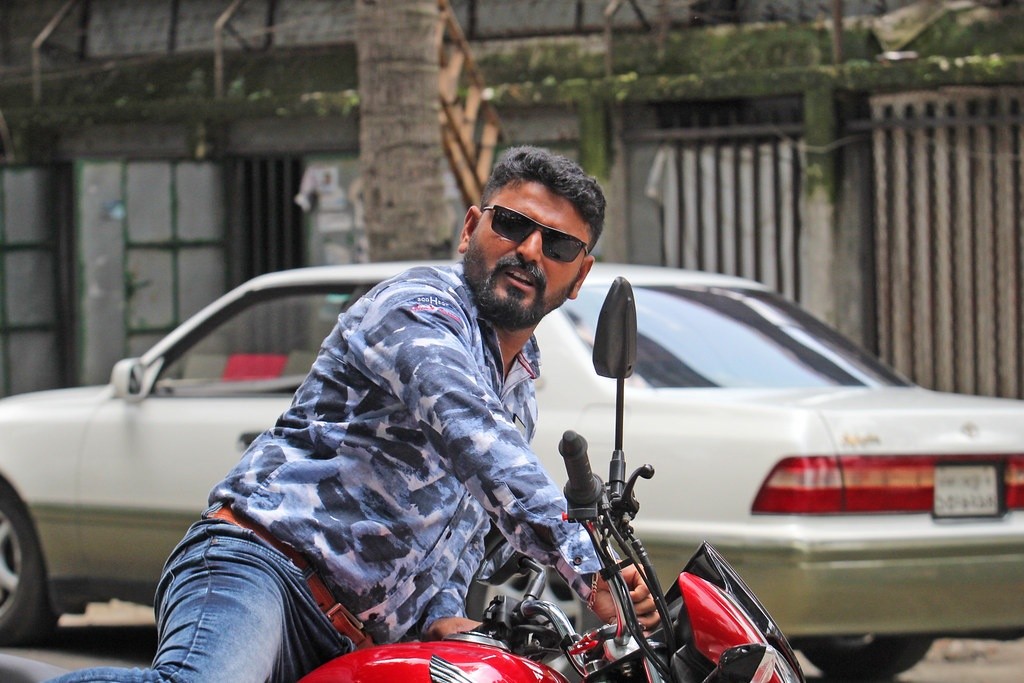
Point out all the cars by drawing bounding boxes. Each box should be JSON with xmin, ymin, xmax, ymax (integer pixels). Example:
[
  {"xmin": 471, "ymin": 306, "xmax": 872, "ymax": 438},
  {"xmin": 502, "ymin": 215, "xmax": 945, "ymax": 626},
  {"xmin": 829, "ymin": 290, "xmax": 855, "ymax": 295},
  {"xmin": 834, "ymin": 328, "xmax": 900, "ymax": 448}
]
[{"xmin": 2, "ymin": 259, "xmax": 1024, "ymax": 678}]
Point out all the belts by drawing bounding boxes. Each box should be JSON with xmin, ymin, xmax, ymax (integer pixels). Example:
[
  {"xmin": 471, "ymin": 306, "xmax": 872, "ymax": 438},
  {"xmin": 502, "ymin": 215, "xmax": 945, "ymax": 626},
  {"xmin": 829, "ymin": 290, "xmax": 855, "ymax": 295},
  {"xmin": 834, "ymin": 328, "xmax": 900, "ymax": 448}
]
[{"xmin": 206, "ymin": 502, "xmax": 374, "ymax": 648}]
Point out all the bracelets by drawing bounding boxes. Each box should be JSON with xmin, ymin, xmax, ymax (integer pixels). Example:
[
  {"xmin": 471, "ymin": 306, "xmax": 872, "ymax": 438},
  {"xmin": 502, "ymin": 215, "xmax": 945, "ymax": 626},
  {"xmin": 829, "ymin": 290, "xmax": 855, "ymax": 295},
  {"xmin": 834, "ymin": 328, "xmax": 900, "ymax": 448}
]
[{"xmin": 587, "ymin": 573, "xmax": 598, "ymax": 611}]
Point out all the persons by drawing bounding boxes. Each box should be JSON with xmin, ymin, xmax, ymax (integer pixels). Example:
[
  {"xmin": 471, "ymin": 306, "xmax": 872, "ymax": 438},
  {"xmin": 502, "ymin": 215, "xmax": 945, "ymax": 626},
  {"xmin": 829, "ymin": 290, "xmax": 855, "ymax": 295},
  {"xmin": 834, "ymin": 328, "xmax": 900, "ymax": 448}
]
[{"xmin": 46, "ymin": 146, "xmax": 661, "ymax": 683}]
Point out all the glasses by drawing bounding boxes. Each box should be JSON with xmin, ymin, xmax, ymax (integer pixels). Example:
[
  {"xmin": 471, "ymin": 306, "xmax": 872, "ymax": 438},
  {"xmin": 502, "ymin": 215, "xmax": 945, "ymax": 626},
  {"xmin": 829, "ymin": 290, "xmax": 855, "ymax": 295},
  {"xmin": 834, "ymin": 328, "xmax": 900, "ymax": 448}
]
[{"xmin": 483, "ymin": 205, "xmax": 589, "ymax": 262}]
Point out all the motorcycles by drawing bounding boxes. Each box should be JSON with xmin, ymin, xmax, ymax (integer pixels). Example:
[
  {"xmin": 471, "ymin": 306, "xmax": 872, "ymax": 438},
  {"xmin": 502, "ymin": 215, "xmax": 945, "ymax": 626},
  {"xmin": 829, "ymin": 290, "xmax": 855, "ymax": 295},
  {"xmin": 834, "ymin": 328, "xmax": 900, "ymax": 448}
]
[{"xmin": 1, "ymin": 275, "xmax": 811, "ymax": 683}]
